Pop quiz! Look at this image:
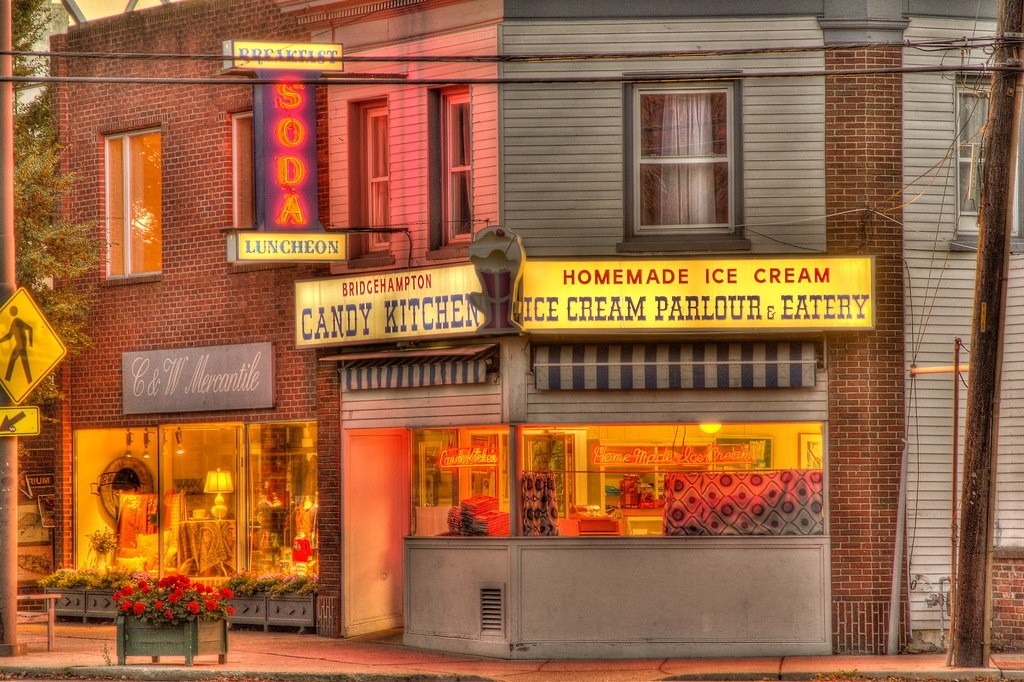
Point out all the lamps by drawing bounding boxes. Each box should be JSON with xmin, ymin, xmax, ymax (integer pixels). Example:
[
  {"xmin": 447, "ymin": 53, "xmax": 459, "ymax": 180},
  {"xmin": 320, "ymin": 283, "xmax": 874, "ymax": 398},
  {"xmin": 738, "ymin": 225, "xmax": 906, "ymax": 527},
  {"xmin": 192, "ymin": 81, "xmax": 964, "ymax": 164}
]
[
  {"xmin": 699, "ymin": 424, "xmax": 721, "ymax": 433},
  {"xmin": 124, "ymin": 428, "xmax": 134, "ymax": 457},
  {"xmin": 142, "ymin": 428, "xmax": 150, "ymax": 458},
  {"xmin": 204, "ymin": 469, "xmax": 234, "ymax": 520},
  {"xmin": 176, "ymin": 428, "xmax": 184, "ymax": 454}
]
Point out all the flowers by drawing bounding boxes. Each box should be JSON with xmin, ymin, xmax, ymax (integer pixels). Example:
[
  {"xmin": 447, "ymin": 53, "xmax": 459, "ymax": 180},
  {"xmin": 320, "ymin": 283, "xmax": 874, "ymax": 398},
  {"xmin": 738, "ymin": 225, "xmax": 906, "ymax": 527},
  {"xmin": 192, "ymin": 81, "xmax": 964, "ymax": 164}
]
[
  {"xmin": 86, "ymin": 526, "xmax": 121, "ymax": 555},
  {"xmin": 227, "ymin": 571, "xmax": 319, "ymax": 595},
  {"xmin": 36, "ymin": 568, "xmax": 151, "ymax": 590},
  {"xmin": 114, "ymin": 575, "xmax": 236, "ymax": 625}
]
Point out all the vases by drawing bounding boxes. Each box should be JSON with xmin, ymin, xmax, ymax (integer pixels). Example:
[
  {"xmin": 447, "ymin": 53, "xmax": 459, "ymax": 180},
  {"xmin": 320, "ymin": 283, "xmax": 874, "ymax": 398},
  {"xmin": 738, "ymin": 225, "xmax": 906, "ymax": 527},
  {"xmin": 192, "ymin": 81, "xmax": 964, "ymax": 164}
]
[
  {"xmin": 95, "ymin": 550, "xmax": 108, "ymax": 577},
  {"xmin": 226, "ymin": 593, "xmax": 316, "ymax": 632},
  {"xmin": 46, "ymin": 588, "xmax": 118, "ymax": 624},
  {"xmin": 115, "ymin": 616, "xmax": 228, "ymax": 667}
]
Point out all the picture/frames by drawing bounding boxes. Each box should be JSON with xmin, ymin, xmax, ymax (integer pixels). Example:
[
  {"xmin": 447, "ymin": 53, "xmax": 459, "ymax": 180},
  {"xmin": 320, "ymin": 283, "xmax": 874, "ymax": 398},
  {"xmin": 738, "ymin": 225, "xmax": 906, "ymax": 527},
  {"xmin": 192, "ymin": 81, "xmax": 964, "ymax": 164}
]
[{"xmin": 797, "ymin": 432, "xmax": 823, "ymax": 469}]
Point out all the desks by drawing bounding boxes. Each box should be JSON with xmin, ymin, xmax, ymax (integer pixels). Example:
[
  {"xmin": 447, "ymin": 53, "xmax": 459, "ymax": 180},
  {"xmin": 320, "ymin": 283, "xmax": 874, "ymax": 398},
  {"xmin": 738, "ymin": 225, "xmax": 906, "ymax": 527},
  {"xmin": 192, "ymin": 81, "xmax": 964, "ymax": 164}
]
[{"xmin": 179, "ymin": 520, "xmax": 237, "ymax": 576}]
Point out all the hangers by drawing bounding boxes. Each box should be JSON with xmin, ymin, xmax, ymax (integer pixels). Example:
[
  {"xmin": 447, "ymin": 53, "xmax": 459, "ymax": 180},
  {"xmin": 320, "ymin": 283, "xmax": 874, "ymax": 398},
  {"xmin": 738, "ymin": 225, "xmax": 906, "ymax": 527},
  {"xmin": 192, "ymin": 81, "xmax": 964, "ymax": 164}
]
[{"xmin": 523, "ymin": 469, "xmax": 555, "ymax": 477}]
